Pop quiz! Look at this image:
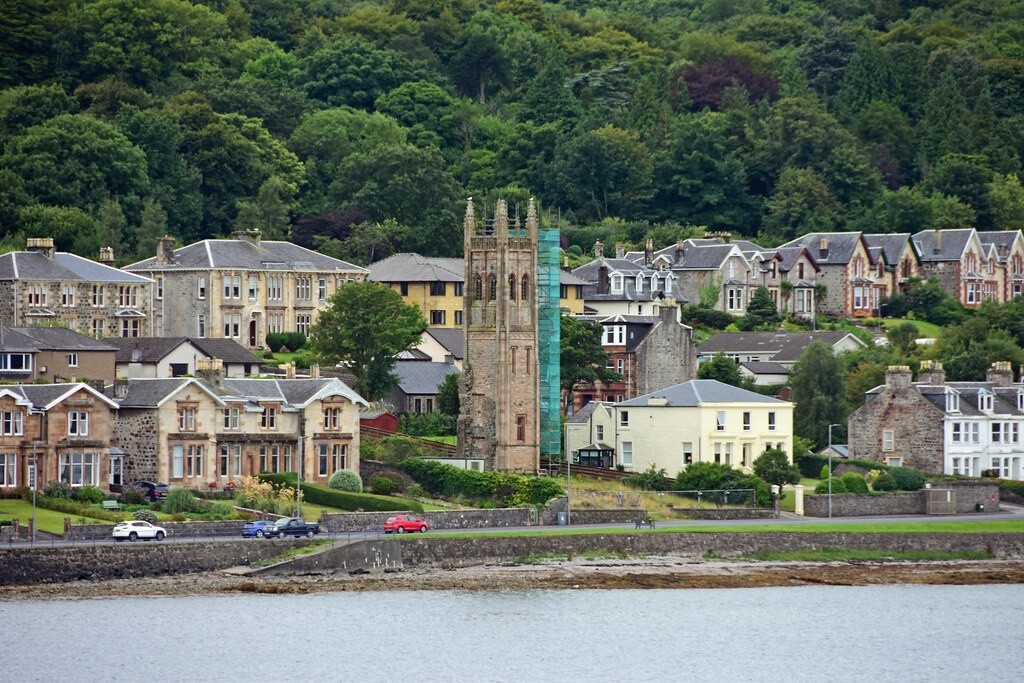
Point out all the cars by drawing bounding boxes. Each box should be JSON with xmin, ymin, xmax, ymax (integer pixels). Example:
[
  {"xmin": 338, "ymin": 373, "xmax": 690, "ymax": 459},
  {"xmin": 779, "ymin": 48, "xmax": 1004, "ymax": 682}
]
[
  {"xmin": 241, "ymin": 521, "xmax": 279, "ymax": 538},
  {"xmin": 112, "ymin": 519, "xmax": 167, "ymax": 542},
  {"xmin": 384, "ymin": 514, "xmax": 428, "ymax": 533}
]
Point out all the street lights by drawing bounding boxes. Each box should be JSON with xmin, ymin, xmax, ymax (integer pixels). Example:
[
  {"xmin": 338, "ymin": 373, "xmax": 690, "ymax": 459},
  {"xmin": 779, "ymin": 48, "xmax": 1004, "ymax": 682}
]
[
  {"xmin": 828, "ymin": 423, "xmax": 843, "ymax": 518},
  {"xmin": 296, "ymin": 435, "xmax": 311, "ymax": 518},
  {"xmin": 31, "ymin": 440, "xmax": 44, "ymax": 543}
]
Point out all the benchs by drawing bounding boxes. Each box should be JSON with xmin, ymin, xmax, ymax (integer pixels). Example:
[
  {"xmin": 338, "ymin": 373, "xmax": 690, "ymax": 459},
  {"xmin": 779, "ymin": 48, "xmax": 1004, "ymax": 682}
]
[
  {"xmin": 633, "ymin": 512, "xmax": 655, "ymax": 529},
  {"xmin": 102, "ymin": 500, "xmax": 122, "ymax": 512}
]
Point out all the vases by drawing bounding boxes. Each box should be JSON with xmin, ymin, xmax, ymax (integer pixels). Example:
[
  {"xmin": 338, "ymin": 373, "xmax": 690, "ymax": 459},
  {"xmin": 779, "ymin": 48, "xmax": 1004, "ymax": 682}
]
[{"xmin": 210, "ymin": 487, "xmax": 216, "ymax": 493}]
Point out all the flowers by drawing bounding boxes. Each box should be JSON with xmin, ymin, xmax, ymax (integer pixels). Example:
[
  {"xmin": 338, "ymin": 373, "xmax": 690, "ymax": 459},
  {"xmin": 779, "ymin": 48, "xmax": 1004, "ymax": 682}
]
[{"xmin": 209, "ymin": 482, "xmax": 217, "ymax": 487}]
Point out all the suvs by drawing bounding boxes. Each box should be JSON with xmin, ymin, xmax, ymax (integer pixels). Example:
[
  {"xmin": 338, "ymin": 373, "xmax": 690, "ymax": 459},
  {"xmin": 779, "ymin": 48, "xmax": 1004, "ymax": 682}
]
[{"xmin": 121, "ymin": 480, "xmax": 173, "ymax": 504}]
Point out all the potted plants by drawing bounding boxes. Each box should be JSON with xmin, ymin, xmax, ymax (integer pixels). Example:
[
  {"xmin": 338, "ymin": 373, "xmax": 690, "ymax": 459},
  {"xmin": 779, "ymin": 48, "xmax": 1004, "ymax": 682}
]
[{"xmin": 114, "ymin": 376, "xmax": 128, "ymax": 399}]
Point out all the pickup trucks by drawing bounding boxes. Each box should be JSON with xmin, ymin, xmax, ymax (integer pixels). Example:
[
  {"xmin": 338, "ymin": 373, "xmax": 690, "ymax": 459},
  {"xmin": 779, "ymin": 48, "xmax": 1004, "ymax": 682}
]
[{"xmin": 264, "ymin": 517, "xmax": 322, "ymax": 538}]
